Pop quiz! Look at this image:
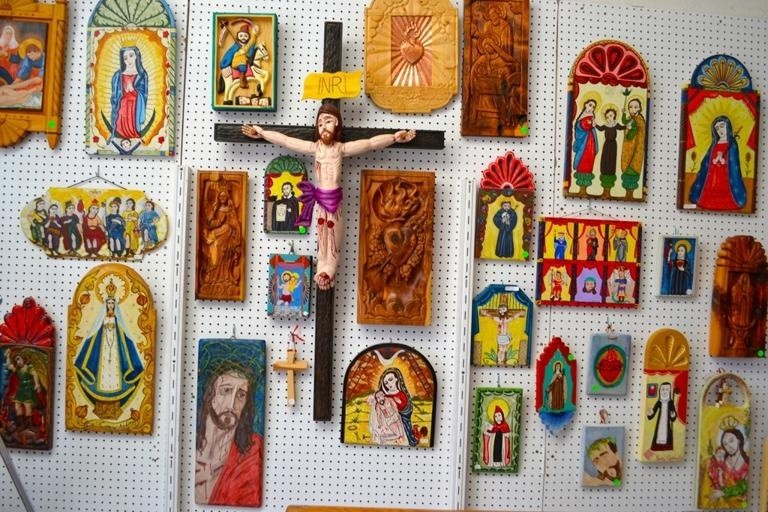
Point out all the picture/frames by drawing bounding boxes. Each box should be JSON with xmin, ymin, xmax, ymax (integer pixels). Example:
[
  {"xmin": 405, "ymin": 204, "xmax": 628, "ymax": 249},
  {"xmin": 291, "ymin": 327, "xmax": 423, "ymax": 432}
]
[{"xmin": 0, "ymin": 0, "xmax": 69, "ymax": 150}]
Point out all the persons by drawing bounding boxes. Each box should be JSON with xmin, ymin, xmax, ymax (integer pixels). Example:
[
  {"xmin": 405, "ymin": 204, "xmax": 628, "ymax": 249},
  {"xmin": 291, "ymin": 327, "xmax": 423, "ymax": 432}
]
[
  {"xmin": 195, "ymin": 361, "xmax": 262, "ymax": 506},
  {"xmin": 469, "ymin": 5, "xmax": 514, "ymax": 126},
  {"xmin": 4, "ymin": 349, "xmax": 41, "ymax": 433},
  {"xmin": 726, "ymin": 272, "xmax": 754, "ymax": 328},
  {"xmin": 206, "ymin": 184, "xmax": 240, "ymax": 285},
  {"xmin": 241, "ymin": 103, "xmax": 415, "ymax": 291}
]
